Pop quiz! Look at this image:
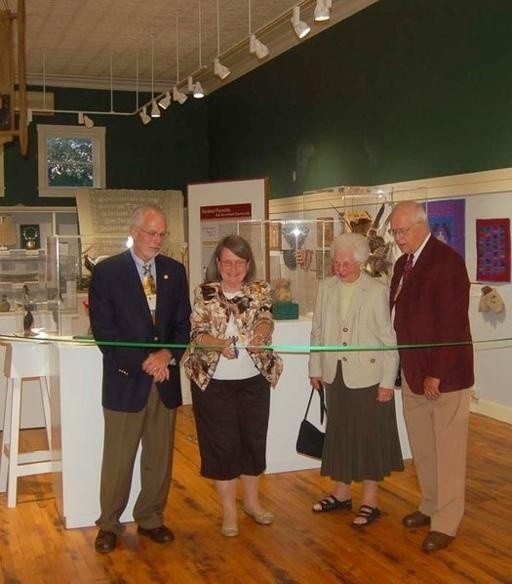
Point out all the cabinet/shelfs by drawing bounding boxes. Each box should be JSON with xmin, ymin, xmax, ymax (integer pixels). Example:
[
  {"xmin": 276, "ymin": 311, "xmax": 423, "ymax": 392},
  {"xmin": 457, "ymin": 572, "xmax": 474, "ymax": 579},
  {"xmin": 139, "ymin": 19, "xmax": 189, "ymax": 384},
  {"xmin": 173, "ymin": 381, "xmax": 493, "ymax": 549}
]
[{"xmin": 1, "ymin": 206, "xmax": 83, "ymax": 315}]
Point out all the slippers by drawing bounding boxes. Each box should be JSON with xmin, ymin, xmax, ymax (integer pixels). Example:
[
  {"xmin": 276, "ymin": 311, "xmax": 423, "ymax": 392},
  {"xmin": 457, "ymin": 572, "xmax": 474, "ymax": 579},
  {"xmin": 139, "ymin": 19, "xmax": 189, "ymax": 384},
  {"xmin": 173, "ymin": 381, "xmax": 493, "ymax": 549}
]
[
  {"xmin": 352, "ymin": 505, "xmax": 380, "ymax": 526},
  {"xmin": 313, "ymin": 495, "xmax": 351, "ymax": 512}
]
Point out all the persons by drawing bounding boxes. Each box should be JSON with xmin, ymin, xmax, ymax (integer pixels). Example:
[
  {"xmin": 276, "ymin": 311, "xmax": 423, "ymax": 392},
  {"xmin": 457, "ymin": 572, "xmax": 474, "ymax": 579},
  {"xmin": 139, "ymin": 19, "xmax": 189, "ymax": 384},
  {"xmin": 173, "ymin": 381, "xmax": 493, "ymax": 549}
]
[
  {"xmin": 88, "ymin": 207, "xmax": 192, "ymax": 553},
  {"xmin": 389, "ymin": 200, "xmax": 474, "ymax": 552},
  {"xmin": 182, "ymin": 235, "xmax": 284, "ymax": 538},
  {"xmin": 307, "ymin": 233, "xmax": 406, "ymax": 529}
]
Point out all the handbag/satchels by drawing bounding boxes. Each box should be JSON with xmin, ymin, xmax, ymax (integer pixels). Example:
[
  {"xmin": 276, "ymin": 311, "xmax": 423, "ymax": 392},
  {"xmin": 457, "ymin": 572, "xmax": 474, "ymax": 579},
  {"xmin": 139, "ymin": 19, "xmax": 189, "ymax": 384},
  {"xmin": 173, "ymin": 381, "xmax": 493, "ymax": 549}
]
[{"xmin": 297, "ymin": 420, "xmax": 326, "ymax": 458}]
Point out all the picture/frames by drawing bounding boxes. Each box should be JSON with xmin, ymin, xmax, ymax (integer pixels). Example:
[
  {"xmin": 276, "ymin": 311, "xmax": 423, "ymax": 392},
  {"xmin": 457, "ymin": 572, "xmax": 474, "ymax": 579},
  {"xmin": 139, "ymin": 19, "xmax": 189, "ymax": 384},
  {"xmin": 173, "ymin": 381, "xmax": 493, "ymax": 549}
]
[{"xmin": 37, "ymin": 123, "xmax": 108, "ymax": 197}]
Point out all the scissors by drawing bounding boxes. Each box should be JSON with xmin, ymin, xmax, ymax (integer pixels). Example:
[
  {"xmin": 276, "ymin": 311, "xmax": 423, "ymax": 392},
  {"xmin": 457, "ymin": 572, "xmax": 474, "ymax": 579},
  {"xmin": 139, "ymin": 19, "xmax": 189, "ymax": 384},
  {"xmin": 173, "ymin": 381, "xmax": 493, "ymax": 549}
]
[{"xmin": 232, "ymin": 335, "xmax": 240, "ymax": 359}]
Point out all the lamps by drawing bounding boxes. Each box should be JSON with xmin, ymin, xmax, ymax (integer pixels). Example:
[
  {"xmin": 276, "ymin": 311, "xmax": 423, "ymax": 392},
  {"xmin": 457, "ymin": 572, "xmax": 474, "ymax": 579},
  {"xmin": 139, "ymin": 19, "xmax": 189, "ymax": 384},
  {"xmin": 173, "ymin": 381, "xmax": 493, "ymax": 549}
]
[
  {"xmin": 78, "ymin": 111, "xmax": 97, "ymax": 130},
  {"xmin": 139, "ymin": 1, "xmax": 333, "ymax": 126}
]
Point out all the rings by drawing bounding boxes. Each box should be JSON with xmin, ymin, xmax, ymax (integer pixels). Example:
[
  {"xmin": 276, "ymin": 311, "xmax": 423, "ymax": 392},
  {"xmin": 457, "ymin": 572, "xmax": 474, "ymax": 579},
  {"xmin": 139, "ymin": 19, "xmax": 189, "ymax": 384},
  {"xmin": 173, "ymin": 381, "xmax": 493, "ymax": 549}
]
[{"xmin": 154, "ymin": 368, "xmax": 156, "ymax": 371}]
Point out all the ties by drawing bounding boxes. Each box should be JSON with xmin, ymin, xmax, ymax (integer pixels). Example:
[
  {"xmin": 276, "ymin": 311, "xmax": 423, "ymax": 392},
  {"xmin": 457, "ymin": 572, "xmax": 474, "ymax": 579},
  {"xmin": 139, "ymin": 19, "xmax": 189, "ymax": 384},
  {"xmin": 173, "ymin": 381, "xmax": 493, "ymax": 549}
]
[{"xmin": 143, "ymin": 263, "xmax": 156, "ymax": 326}]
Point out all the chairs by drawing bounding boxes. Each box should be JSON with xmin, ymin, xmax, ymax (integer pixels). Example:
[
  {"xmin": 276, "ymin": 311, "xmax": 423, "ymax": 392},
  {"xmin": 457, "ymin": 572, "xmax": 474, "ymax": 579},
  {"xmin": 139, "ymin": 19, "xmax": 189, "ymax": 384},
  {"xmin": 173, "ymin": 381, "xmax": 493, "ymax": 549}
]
[{"xmin": 1, "ymin": 339, "xmax": 55, "ymax": 510}]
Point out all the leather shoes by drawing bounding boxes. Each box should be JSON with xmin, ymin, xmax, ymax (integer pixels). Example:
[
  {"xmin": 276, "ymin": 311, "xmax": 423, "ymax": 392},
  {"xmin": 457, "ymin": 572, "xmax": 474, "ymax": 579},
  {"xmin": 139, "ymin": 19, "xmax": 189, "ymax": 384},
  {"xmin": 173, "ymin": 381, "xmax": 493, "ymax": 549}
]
[
  {"xmin": 221, "ymin": 527, "xmax": 240, "ymax": 537},
  {"xmin": 138, "ymin": 525, "xmax": 174, "ymax": 543},
  {"xmin": 95, "ymin": 530, "xmax": 115, "ymax": 552},
  {"xmin": 241, "ymin": 503, "xmax": 273, "ymax": 524},
  {"xmin": 422, "ymin": 532, "xmax": 456, "ymax": 550},
  {"xmin": 403, "ymin": 512, "xmax": 431, "ymax": 526}
]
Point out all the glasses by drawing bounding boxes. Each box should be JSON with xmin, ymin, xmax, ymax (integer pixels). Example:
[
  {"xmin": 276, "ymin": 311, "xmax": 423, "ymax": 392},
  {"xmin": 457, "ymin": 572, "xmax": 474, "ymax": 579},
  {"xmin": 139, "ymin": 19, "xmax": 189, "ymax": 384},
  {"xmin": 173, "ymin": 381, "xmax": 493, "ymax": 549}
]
[
  {"xmin": 141, "ymin": 229, "xmax": 170, "ymax": 239},
  {"xmin": 388, "ymin": 225, "xmax": 410, "ymax": 237}
]
